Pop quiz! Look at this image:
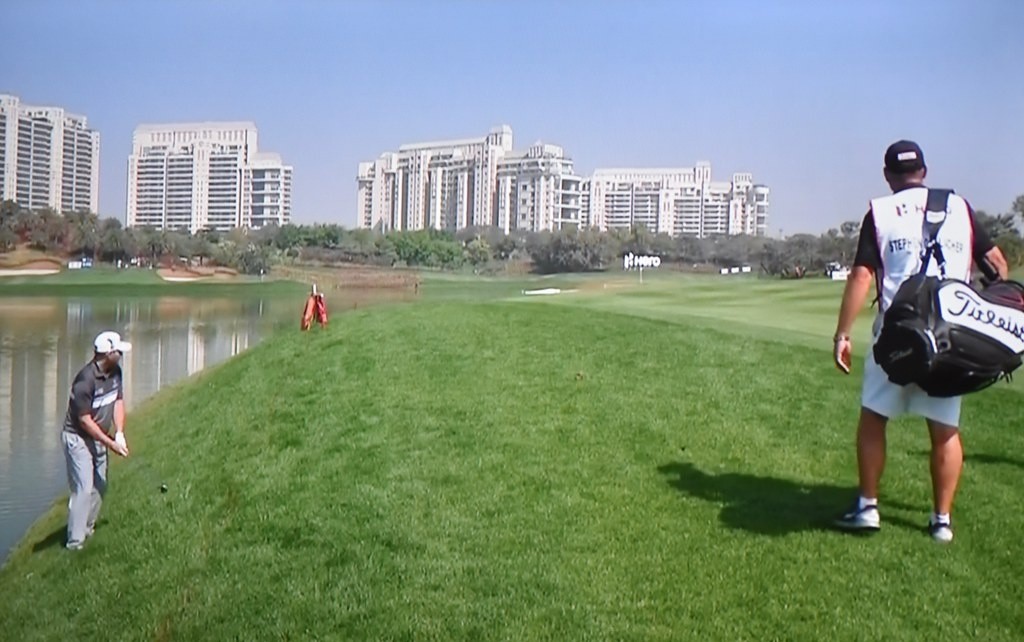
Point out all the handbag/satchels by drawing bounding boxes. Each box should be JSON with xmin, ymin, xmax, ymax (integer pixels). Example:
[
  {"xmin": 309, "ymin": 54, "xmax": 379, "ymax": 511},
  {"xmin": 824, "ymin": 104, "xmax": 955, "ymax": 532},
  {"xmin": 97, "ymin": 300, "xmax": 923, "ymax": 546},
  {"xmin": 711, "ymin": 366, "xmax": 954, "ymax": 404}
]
[{"xmin": 871, "ymin": 270, "xmax": 1024, "ymax": 397}]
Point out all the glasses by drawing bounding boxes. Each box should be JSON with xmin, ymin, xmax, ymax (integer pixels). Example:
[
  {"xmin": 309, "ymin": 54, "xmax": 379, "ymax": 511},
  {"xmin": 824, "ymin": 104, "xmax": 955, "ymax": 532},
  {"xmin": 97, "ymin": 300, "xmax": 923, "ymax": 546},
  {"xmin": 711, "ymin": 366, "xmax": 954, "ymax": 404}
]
[{"xmin": 116, "ymin": 350, "xmax": 123, "ymax": 355}]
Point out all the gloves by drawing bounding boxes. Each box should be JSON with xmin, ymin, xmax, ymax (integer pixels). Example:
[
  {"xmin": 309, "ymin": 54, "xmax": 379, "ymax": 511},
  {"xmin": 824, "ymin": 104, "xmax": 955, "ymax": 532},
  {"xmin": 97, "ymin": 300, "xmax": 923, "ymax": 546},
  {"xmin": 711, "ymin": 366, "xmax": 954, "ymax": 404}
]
[{"xmin": 114, "ymin": 432, "xmax": 126, "ymax": 449}]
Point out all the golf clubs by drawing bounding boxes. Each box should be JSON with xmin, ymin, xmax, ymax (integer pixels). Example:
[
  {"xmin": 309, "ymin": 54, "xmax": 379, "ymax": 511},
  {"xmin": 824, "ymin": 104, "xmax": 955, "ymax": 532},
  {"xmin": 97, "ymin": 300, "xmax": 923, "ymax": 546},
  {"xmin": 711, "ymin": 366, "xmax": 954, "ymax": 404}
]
[{"xmin": 128, "ymin": 452, "xmax": 170, "ymax": 494}]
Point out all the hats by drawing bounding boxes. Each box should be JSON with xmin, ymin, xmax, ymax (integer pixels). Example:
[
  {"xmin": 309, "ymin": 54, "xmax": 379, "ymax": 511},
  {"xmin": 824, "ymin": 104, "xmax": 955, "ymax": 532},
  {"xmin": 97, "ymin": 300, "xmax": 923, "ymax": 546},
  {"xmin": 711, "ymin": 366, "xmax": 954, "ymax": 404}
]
[
  {"xmin": 93, "ymin": 331, "xmax": 132, "ymax": 353},
  {"xmin": 885, "ymin": 140, "xmax": 923, "ymax": 167}
]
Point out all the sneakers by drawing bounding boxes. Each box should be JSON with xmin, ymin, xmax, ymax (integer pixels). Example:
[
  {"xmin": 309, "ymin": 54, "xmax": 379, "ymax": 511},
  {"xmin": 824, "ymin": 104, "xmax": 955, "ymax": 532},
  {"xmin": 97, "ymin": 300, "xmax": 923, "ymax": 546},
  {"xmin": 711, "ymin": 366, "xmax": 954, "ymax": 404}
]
[
  {"xmin": 833, "ymin": 504, "xmax": 880, "ymax": 530},
  {"xmin": 929, "ymin": 518, "xmax": 954, "ymax": 541}
]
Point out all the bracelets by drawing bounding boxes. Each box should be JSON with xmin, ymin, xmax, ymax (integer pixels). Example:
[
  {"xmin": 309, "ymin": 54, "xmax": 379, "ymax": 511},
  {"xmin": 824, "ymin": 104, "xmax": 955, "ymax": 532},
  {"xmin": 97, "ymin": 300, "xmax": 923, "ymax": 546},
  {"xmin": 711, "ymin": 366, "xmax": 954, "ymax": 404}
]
[{"xmin": 833, "ymin": 334, "xmax": 850, "ymax": 343}]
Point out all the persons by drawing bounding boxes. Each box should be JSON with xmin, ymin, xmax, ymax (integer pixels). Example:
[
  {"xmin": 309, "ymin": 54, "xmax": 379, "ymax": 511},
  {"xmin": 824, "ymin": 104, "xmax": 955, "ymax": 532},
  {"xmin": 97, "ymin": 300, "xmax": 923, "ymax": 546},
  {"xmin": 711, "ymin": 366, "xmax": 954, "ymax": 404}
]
[
  {"xmin": 831, "ymin": 142, "xmax": 1007, "ymax": 544},
  {"xmin": 61, "ymin": 331, "xmax": 132, "ymax": 552}
]
